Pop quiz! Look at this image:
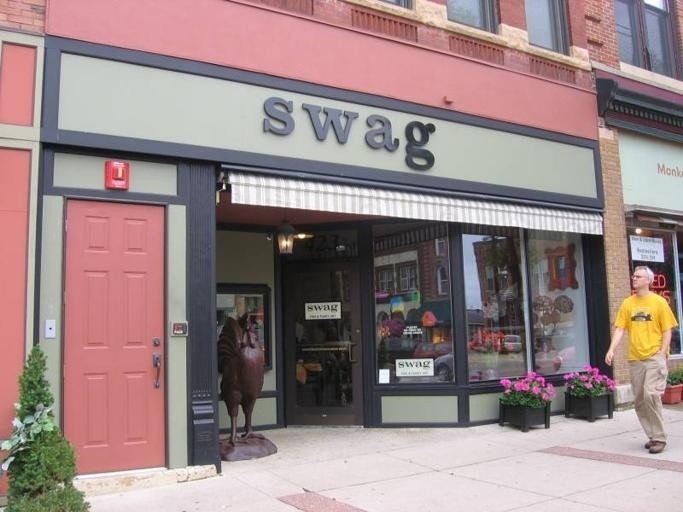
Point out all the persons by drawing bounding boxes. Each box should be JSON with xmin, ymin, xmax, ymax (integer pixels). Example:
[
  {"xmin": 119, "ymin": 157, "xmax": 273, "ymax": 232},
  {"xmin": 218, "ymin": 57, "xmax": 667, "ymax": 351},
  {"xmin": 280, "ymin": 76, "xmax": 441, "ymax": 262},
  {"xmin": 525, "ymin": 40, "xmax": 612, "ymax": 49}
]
[{"xmin": 605, "ymin": 266, "xmax": 678, "ymax": 453}]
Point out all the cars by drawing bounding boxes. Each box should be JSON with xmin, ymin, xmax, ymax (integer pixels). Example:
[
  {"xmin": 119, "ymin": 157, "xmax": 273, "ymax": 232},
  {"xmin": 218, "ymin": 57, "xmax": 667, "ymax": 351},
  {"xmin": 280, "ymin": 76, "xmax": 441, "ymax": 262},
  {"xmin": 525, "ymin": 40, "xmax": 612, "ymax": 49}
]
[{"xmin": 388, "ymin": 334, "xmax": 590, "ymax": 381}]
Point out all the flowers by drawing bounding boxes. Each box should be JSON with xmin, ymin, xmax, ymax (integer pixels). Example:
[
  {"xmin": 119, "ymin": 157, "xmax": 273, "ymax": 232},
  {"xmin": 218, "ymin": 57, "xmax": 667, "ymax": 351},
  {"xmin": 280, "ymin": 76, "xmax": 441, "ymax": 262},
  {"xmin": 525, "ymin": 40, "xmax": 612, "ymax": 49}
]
[
  {"xmin": 562, "ymin": 366, "xmax": 617, "ymax": 397},
  {"xmin": 499, "ymin": 370, "xmax": 556, "ymax": 410}
]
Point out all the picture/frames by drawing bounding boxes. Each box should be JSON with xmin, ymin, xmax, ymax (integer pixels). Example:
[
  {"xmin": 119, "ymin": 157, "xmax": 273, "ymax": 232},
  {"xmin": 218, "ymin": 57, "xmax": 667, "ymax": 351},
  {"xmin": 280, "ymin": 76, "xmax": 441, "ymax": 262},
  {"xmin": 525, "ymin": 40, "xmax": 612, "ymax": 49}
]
[{"xmin": 544, "ymin": 243, "xmax": 578, "ymax": 291}]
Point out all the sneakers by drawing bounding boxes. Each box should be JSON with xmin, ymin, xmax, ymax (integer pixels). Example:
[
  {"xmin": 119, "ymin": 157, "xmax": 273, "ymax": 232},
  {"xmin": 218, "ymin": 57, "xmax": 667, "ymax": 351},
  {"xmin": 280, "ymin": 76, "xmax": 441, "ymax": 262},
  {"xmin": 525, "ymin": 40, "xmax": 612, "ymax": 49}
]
[{"xmin": 645, "ymin": 441, "xmax": 666, "ymax": 454}]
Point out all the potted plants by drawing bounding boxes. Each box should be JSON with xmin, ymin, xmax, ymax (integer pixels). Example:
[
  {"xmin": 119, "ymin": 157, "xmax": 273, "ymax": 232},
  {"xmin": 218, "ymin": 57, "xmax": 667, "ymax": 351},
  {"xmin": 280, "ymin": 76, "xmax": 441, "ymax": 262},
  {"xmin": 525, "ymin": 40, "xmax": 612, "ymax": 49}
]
[{"xmin": 663, "ymin": 367, "xmax": 683, "ymax": 405}]
